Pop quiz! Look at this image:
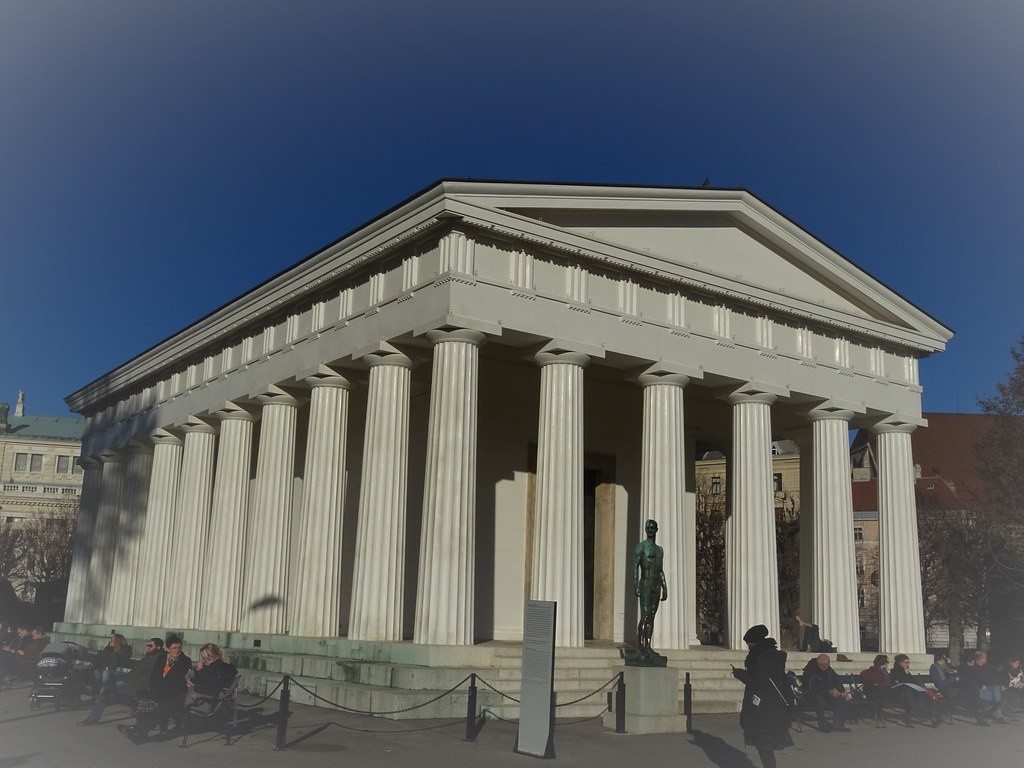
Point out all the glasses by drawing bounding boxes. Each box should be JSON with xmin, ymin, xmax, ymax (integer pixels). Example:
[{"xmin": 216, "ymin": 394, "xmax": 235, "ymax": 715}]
[
  {"xmin": 886, "ymin": 660, "xmax": 890, "ymax": 664},
  {"xmin": 938, "ymin": 658, "xmax": 946, "ymax": 661},
  {"xmin": 147, "ymin": 644, "xmax": 154, "ymax": 648},
  {"xmin": 903, "ymin": 661, "xmax": 911, "ymax": 665},
  {"xmin": 111, "ymin": 638, "xmax": 114, "ymax": 643},
  {"xmin": 169, "ymin": 647, "xmax": 182, "ymax": 650}
]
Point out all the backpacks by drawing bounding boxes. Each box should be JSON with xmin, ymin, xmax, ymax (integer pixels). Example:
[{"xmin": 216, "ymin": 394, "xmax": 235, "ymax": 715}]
[{"xmin": 768, "ymin": 671, "xmax": 807, "ymax": 712}]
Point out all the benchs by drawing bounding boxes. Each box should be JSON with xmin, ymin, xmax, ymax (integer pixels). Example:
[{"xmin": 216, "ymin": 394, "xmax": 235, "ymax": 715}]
[
  {"xmin": 911, "ymin": 675, "xmax": 954, "ymax": 725},
  {"xmin": 788, "ymin": 672, "xmax": 881, "ymax": 733},
  {"xmin": 73, "ymin": 652, "xmax": 242, "ymax": 749}
]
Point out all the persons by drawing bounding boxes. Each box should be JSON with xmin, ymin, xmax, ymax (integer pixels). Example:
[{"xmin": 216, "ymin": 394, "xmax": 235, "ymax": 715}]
[
  {"xmin": 796, "ymin": 615, "xmax": 833, "ymax": 652},
  {"xmin": 733, "ymin": 625, "xmax": 794, "ymax": 768},
  {"xmin": 77, "ymin": 635, "xmax": 237, "ymax": 745},
  {"xmin": 860, "ymin": 651, "xmax": 1024, "ymax": 726},
  {"xmin": 631, "ymin": 519, "xmax": 668, "ymax": 650},
  {"xmin": 0, "ymin": 621, "xmax": 48, "ymax": 682},
  {"xmin": 802, "ymin": 654, "xmax": 851, "ymax": 732}
]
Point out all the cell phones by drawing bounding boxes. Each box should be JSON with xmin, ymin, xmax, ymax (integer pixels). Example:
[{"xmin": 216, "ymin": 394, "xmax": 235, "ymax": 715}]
[{"xmin": 730, "ymin": 664, "xmax": 735, "ymax": 668}]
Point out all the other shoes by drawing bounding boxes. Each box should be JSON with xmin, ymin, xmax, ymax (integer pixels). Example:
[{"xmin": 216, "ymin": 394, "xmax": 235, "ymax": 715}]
[
  {"xmin": 818, "ymin": 720, "xmax": 852, "ymax": 733},
  {"xmin": 976, "ymin": 719, "xmax": 992, "ymax": 727},
  {"xmin": 117, "ymin": 723, "xmax": 146, "ymax": 745},
  {"xmin": 77, "ymin": 716, "xmax": 99, "ymax": 726},
  {"xmin": 994, "ymin": 717, "xmax": 1010, "ymax": 724}
]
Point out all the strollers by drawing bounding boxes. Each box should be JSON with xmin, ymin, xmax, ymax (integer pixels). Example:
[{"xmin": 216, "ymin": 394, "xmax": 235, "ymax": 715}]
[{"xmin": 29, "ymin": 640, "xmax": 88, "ymax": 711}]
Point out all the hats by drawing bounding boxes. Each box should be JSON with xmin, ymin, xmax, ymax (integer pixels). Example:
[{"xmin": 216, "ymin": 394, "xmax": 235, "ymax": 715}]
[{"xmin": 743, "ymin": 624, "xmax": 769, "ymax": 642}]
